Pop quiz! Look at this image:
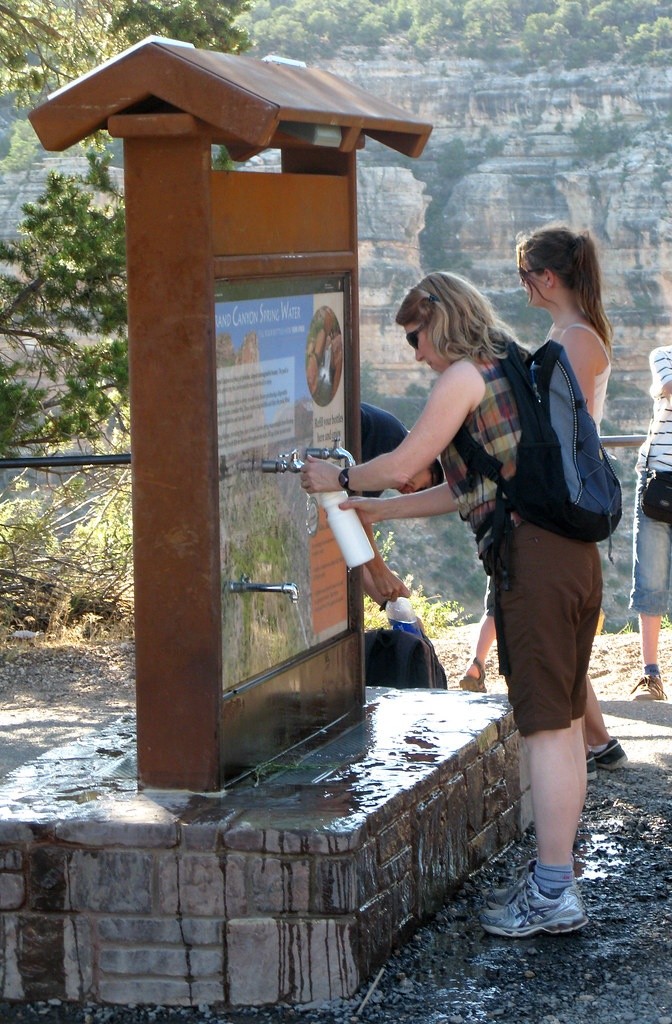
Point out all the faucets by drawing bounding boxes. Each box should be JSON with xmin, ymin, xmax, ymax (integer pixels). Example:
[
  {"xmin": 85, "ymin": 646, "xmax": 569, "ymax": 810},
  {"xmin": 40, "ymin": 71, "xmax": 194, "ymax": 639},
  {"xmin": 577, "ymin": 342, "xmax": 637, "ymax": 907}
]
[
  {"xmin": 260, "ymin": 448, "xmax": 322, "ymax": 473},
  {"xmin": 306, "ymin": 437, "xmax": 357, "ymax": 468},
  {"xmin": 242, "ymin": 582, "xmax": 301, "ymax": 606}
]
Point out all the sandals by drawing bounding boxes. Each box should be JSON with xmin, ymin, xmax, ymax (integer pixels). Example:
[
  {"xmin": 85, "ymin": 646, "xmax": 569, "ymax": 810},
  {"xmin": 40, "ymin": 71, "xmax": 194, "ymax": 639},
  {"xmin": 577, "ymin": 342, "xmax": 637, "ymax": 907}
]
[{"xmin": 461, "ymin": 659, "xmax": 488, "ymax": 694}]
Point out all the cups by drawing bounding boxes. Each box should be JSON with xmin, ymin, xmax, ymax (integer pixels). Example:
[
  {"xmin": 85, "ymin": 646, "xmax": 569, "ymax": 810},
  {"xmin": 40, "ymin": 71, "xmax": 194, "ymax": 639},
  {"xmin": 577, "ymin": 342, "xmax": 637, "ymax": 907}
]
[{"xmin": 320, "ymin": 488, "xmax": 374, "ymax": 570}]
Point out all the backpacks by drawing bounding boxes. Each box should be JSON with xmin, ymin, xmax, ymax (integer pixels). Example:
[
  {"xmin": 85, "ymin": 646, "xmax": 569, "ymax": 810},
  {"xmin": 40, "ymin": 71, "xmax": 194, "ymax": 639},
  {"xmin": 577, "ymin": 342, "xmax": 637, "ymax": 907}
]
[
  {"xmin": 364, "ymin": 623, "xmax": 447, "ymax": 691},
  {"xmin": 499, "ymin": 337, "xmax": 621, "ymax": 538}
]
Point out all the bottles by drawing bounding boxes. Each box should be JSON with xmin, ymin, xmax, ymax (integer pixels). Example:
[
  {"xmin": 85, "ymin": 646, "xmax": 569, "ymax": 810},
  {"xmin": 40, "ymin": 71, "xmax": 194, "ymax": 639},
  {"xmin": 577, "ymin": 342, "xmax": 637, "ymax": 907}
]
[{"xmin": 386, "ymin": 592, "xmax": 424, "ymax": 644}]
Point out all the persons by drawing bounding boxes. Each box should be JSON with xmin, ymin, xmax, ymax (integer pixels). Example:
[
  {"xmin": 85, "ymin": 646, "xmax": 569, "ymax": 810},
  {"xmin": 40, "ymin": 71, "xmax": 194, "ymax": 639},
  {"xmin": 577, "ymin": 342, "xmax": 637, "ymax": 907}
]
[
  {"xmin": 629, "ymin": 344, "xmax": 672, "ymax": 700},
  {"xmin": 361, "ymin": 400, "xmax": 445, "ymax": 613},
  {"xmin": 299, "ymin": 271, "xmax": 604, "ymax": 939},
  {"xmin": 458, "ymin": 574, "xmax": 496, "ymax": 692},
  {"xmin": 515, "ymin": 223, "xmax": 628, "ymax": 782}
]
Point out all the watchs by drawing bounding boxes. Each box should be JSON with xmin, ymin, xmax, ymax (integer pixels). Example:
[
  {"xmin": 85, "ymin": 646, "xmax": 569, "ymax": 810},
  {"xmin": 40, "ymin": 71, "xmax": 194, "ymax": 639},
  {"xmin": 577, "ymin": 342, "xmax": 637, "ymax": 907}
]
[
  {"xmin": 379, "ymin": 599, "xmax": 390, "ymax": 612},
  {"xmin": 339, "ymin": 466, "xmax": 356, "ymax": 494}
]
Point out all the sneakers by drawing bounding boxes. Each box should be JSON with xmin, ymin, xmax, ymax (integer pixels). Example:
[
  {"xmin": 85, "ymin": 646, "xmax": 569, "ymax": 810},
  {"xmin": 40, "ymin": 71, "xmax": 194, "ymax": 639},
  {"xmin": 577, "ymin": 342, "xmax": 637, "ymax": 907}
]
[
  {"xmin": 586, "ymin": 751, "xmax": 598, "ymax": 781},
  {"xmin": 481, "ymin": 886, "xmax": 587, "ymax": 936},
  {"xmin": 591, "ymin": 740, "xmax": 628, "ymax": 771},
  {"xmin": 484, "ymin": 856, "xmax": 542, "ymax": 909},
  {"xmin": 635, "ymin": 674, "xmax": 665, "ymax": 699}
]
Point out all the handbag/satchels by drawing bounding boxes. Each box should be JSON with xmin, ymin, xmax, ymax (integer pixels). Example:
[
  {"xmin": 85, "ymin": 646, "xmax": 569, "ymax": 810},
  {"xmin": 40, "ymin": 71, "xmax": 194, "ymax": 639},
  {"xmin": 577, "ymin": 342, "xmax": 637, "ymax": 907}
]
[{"xmin": 641, "ymin": 468, "xmax": 672, "ymax": 524}]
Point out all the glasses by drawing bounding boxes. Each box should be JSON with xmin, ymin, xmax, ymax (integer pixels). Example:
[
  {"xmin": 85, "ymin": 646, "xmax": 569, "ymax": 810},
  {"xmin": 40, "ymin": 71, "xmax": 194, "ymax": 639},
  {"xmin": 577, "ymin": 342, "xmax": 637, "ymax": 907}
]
[
  {"xmin": 406, "ymin": 320, "xmax": 435, "ymax": 348},
  {"xmin": 518, "ymin": 266, "xmax": 547, "ymax": 279}
]
